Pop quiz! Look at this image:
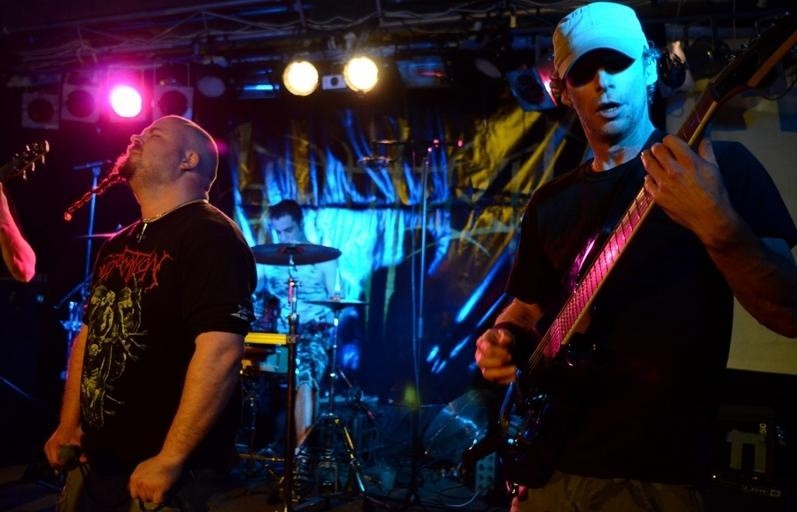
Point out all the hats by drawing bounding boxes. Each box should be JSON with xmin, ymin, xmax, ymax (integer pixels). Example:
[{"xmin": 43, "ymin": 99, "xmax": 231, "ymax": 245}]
[{"xmin": 552, "ymin": 1, "xmax": 650, "ymax": 81}]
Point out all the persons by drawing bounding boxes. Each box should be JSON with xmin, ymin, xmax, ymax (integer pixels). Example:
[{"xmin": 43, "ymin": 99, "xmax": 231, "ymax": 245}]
[
  {"xmin": 359, "ymin": 226, "xmax": 466, "ymax": 406},
  {"xmin": 250, "ymin": 198, "xmax": 337, "ymax": 486},
  {"xmin": 0, "ymin": 181, "xmax": 38, "ymax": 284},
  {"xmin": 40, "ymin": 115, "xmax": 258, "ymax": 511}
]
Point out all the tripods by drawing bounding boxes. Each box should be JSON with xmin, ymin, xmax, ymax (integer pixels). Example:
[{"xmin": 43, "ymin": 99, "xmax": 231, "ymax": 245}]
[{"xmin": 288, "ymin": 354, "xmax": 371, "ymax": 495}]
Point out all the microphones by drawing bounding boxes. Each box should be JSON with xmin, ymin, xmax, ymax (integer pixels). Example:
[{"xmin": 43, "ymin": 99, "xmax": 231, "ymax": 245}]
[{"xmin": 71, "ymin": 157, "xmax": 113, "ymax": 171}]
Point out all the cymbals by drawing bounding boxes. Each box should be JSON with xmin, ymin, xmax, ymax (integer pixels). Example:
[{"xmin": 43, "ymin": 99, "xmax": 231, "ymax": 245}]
[
  {"xmin": 303, "ymin": 296, "xmax": 370, "ymax": 310},
  {"xmin": 252, "ymin": 243, "xmax": 341, "ymax": 267}
]
